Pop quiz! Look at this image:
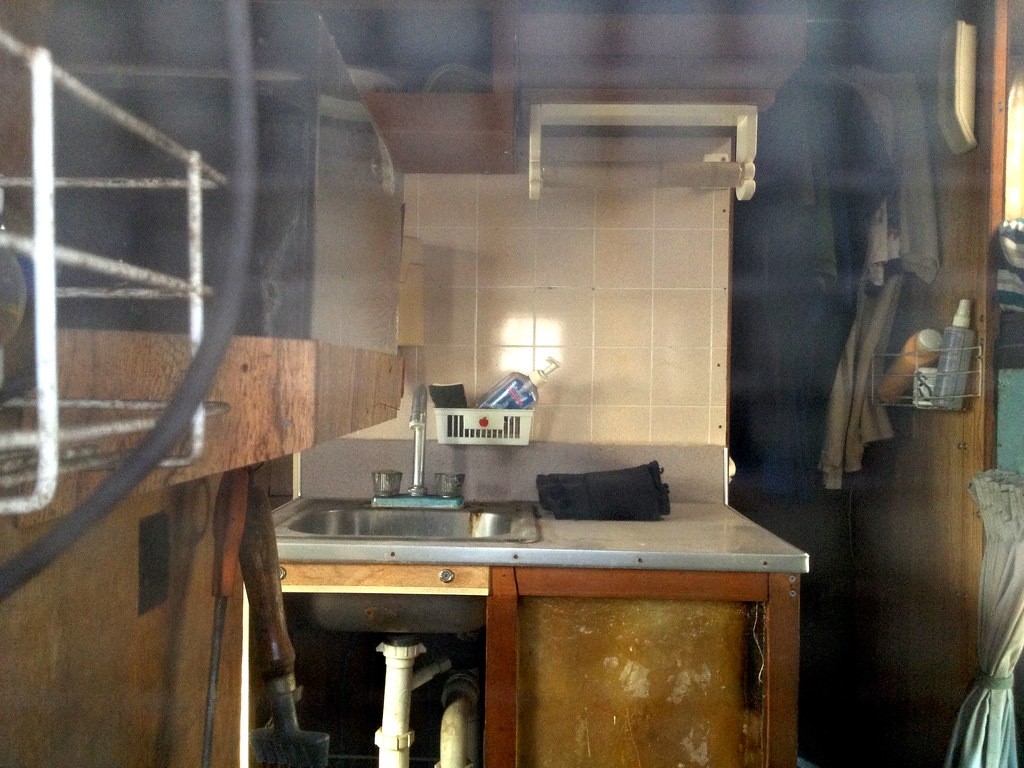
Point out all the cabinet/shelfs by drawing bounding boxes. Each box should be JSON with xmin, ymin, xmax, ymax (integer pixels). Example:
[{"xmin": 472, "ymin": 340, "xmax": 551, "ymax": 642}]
[
  {"xmin": 275, "ymin": 564, "xmax": 799, "ymax": 767},
  {"xmin": 319, "ymin": 2, "xmax": 809, "ymax": 179}
]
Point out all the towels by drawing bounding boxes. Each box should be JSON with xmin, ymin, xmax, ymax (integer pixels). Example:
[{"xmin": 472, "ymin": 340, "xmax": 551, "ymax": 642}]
[
  {"xmin": 816, "ymin": 262, "xmax": 907, "ymax": 497},
  {"xmin": 753, "ymin": 60, "xmax": 943, "ymax": 316},
  {"xmin": 535, "ymin": 459, "xmax": 671, "ymax": 522}
]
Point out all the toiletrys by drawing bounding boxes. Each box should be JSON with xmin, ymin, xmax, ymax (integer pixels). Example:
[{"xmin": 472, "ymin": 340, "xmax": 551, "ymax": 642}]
[{"xmin": 466, "ymin": 357, "xmax": 562, "ymax": 409}]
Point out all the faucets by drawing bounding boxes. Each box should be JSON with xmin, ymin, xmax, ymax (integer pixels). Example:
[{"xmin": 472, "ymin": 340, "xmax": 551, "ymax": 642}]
[{"xmin": 370, "ymin": 383, "xmax": 466, "ymax": 508}]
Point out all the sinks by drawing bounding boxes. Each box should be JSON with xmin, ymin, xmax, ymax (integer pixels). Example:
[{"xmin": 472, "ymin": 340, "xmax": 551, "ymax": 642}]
[{"xmin": 271, "ymin": 497, "xmax": 539, "ymax": 543}]
